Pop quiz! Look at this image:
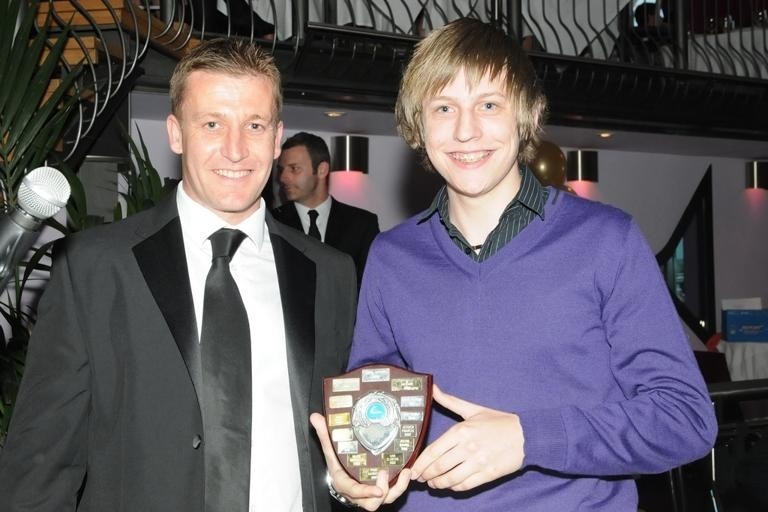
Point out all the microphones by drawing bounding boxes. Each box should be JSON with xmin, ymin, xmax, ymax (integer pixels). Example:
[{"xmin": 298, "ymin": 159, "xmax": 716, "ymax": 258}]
[{"xmin": 0, "ymin": 166, "xmax": 72, "ymax": 296}]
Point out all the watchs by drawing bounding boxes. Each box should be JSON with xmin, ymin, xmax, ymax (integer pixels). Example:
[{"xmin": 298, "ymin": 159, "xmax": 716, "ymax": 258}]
[{"xmin": 323, "ymin": 471, "xmax": 354, "ymax": 508}]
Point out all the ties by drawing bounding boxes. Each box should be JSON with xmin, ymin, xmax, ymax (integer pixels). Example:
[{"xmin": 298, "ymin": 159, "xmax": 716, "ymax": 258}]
[
  {"xmin": 200, "ymin": 228, "xmax": 252, "ymax": 512},
  {"xmin": 308, "ymin": 210, "xmax": 321, "ymax": 242}
]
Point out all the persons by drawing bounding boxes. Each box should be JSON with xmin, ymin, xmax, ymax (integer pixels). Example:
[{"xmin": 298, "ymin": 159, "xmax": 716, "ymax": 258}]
[
  {"xmin": 275, "ymin": 133, "xmax": 382, "ymax": 297},
  {"xmin": 0, "ymin": 39, "xmax": 359, "ymax": 511},
  {"xmin": 307, "ymin": 18, "xmax": 718, "ymax": 512},
  {"xmin": 612, "ymin": 5, "xmax": 678, "ymax": 60}
]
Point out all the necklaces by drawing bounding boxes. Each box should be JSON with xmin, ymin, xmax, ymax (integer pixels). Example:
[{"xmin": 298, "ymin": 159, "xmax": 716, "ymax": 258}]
[{"xmin": 472, "ymin": 245, "xmax": 482, "ymax": 249}]
